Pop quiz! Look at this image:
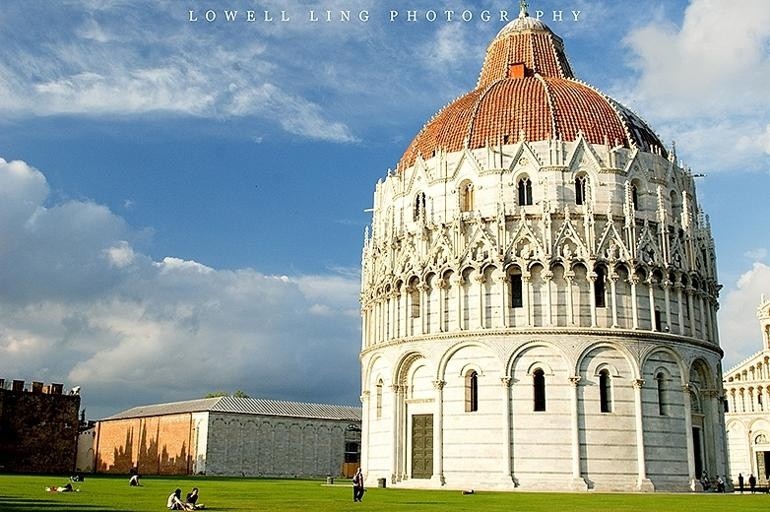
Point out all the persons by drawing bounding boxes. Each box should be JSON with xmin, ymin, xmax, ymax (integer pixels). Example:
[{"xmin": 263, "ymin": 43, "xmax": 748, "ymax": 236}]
[
  {"xmin": 353, "ymin": 467, "xmax": 364, "ymax": 502},
  {"xmin": 738, "ymin": 473, "xmax": 743, "ymax": 493},
  {"xmin": 186, "ymin": 488, "xmax": 204, "ymax": 509},
  {"xmin": 748, "ymin": 474, "xmax": 756, "ymax": 493},
  {"xmin": 167, "ymin": 488, "xmax": 189, "ymax": 510},
  {"xmin": 701, "ymin": 470, "xmax": 725, "ymax": 493},
  {"xmin": 71, "ymin": 471, "xmax": 85, "ymax": 481},
  {"xmin": 129, "ymin": 474, "xmax": 144, "ymax": 487},
  {"xmin": 45, "ymin": 483, "xmax": 73, "ymax": 493}
]
